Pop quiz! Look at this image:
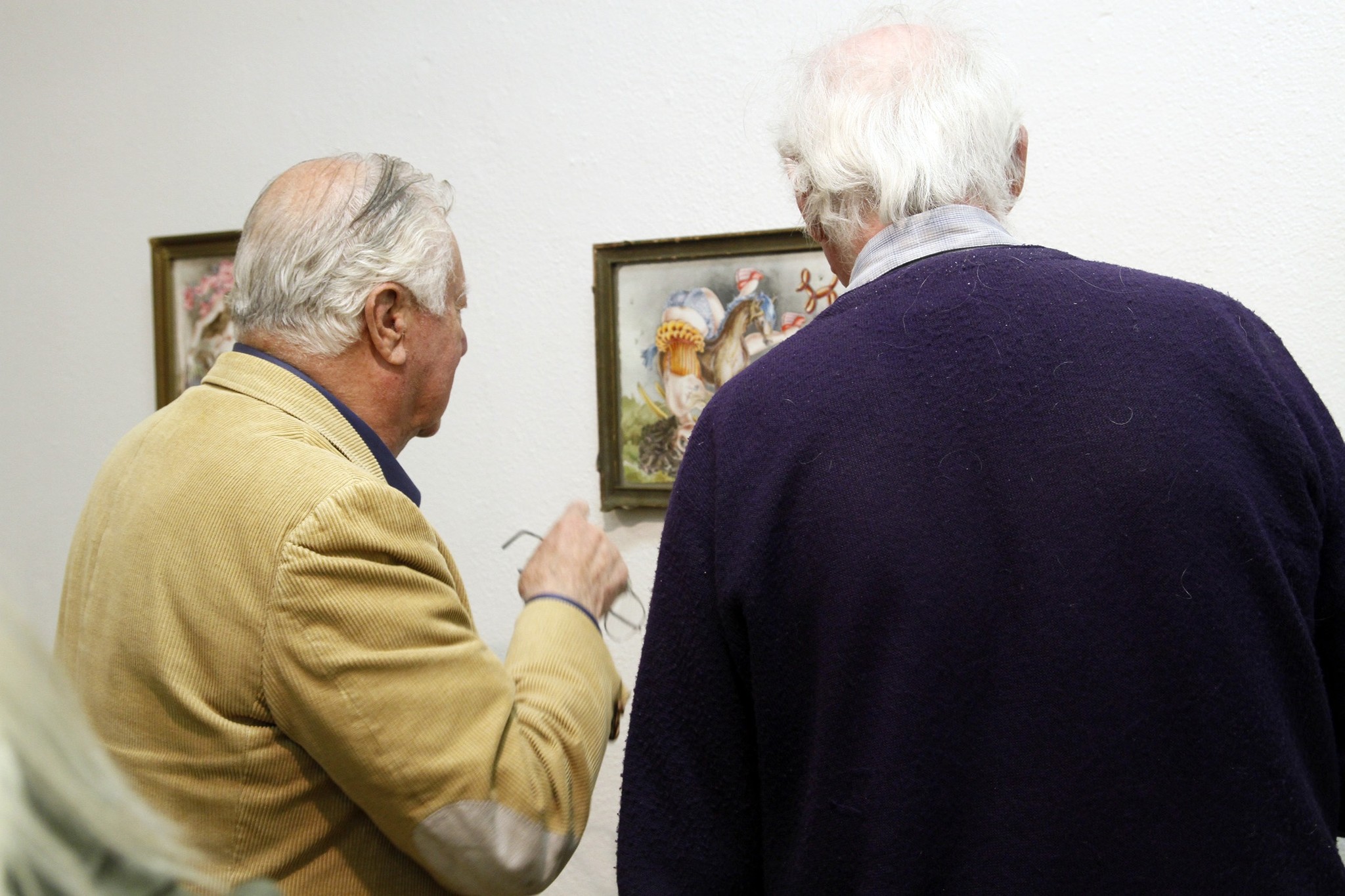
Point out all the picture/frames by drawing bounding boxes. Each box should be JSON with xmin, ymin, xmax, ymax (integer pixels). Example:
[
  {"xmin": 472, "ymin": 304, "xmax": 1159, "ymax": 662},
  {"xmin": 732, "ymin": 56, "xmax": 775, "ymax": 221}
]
[
  {"xmin": 149, "ymin": 230, "xmax": 241, "ymax": 409},
  {"xmin": 592, "ymin": 228, "xmax": 845, "ymax": 512}
]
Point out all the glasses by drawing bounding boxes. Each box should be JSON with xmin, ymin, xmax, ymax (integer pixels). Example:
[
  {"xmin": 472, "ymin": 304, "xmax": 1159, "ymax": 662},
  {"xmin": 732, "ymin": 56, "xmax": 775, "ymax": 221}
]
[{"xmin": 501, "ymin": 531, "xmax": 646, "ymax": 643}]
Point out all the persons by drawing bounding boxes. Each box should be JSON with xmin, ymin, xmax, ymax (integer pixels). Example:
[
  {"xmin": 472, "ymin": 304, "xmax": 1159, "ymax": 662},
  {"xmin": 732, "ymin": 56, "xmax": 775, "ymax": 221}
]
[
  {"xmin": 0, "ymin": 153, "xmax": 635, "ymax": 895},
  {"xmin": 612, "ymin": 21, "xmax": 1345, "ymax": 896}
]
